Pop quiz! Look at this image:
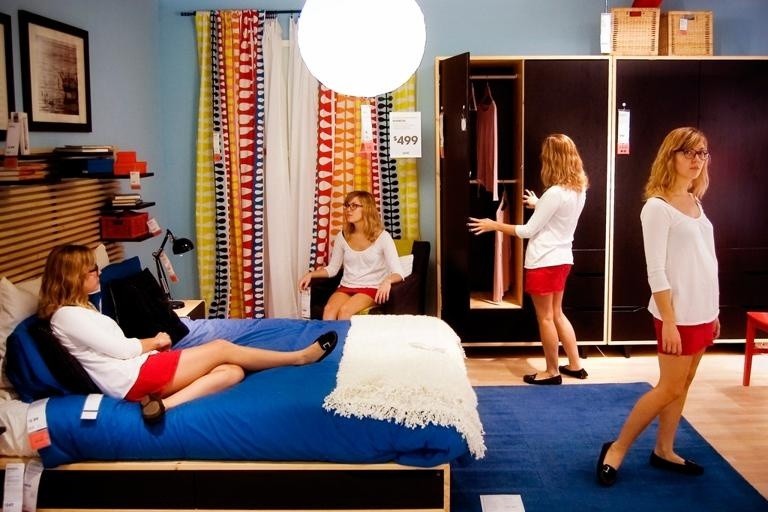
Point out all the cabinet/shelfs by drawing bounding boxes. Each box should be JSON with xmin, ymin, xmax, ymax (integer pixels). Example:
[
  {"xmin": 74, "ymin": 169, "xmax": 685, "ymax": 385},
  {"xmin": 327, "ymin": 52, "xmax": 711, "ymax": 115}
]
[
  {"xmin": 435, "ymin": 56, "xmax": 613, "ymax": 360},
  {"xmin": 609, "ymin": 56, "xmax": 766, "ymax": 362}
]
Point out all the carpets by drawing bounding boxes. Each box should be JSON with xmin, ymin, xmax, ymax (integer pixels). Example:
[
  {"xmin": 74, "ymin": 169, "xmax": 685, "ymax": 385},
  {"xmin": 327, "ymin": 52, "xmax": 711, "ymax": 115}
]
[{"xmin": 450, "ymin": 383, "xmax": 768, "ymax": 511}]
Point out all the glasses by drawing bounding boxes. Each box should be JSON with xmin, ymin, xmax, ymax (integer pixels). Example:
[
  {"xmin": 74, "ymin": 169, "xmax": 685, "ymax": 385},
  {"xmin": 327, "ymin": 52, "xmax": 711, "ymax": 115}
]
[
  {"xmin": 675, "ymin": 148, "xmax": 709, "ymax": 161},
  {"xmin": 343, "ymin": 202, "xmax": 363, "ymax": 211}
]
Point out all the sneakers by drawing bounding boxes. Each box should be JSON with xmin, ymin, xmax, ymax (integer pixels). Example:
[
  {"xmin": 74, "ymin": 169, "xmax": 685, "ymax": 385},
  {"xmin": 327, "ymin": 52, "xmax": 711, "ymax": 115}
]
[
  {"xmin": 139, "ymin": 394, "xmax": 165, "ymax": 423},
  {"xmin": 651, "ymin": 449, "xmax": 704, "ymax": 476},
  {"xmin": 313, "ymin": 331, "xmax": 337, "ymax": 363},
  {"xmin": 524, "ymin": 373, "xmax": 562, "ymax": 385},
  {"xmin": 558, "ymin": 366, "xmax": 588, "ymax": 379},
  {"xmin": 596, "ymin": 442, "xmax": 619, "ymax": 486}
]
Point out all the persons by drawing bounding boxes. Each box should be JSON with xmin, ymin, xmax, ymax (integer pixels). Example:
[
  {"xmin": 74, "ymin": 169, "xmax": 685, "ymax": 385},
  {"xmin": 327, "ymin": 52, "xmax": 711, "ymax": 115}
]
[
  {"xmin": 597, "ymin": 124, "xmax": 723, "ymax": 489},
  {"xmin": 466, "ymin": 132, "xmax": 592, "ymax": 387},
  {"xmin": 298, "ymin": 189, "xmax": 407, "ymax": 322},
  {"xmin": 37, "ymin": 241, "xmax": 339, "ymax": 425}
]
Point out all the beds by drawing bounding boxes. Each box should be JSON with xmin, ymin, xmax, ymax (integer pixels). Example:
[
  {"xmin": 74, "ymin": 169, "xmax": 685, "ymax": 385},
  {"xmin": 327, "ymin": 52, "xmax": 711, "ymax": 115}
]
[{"xmin": 0, "ymin": 309, "xmax": 471, "ymax": 512}]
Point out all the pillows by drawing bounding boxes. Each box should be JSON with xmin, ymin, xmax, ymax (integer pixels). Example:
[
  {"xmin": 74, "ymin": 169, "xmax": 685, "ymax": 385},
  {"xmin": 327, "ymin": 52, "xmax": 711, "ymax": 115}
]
[{"xmin": 1, "ymin": 244, "xmax": 192, "ymax": 396}]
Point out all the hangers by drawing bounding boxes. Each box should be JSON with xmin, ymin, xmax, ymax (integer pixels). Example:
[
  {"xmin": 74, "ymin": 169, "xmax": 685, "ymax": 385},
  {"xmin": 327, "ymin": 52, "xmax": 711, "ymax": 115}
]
[
  {"xmin": 467, "ymin": 73, "xmax": 494, "ymax": 114},
  {"xmin": 495, "ymin": 178, "xmax": 510, "ymax": 212}
]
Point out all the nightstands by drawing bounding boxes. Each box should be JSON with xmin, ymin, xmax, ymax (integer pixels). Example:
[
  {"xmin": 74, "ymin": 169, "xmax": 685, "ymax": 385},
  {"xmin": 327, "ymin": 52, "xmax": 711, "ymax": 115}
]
[{"xmin": 170, "ymin": 298, "xmax": 205, "ymax": 322}]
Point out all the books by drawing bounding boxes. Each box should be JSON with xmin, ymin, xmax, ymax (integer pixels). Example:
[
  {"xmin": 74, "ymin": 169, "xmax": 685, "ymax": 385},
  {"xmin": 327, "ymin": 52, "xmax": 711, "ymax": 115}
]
[{"xmin": 1, "ymin": 107, "xmax": 147, "ymax": 208}]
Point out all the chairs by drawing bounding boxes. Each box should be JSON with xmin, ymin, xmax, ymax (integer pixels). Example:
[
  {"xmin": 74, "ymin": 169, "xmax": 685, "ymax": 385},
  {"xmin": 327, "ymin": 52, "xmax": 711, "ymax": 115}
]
[{"xmin": 312, "ymin": 237, "xmax": 432, "ymax": 320}]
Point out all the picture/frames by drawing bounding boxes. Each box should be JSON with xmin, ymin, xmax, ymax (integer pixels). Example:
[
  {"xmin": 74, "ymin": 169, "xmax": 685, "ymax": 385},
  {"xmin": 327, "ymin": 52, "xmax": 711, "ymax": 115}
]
[{"xmin": 1, "ymin": 9, "xmax": 93, "ymax": 140}]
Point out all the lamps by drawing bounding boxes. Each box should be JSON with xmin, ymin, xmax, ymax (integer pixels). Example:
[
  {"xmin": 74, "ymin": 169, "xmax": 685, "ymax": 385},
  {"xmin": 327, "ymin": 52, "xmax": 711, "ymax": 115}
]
[{"xmin": 153, "ymin": 228, "xmax": 194, "ymax": 311}]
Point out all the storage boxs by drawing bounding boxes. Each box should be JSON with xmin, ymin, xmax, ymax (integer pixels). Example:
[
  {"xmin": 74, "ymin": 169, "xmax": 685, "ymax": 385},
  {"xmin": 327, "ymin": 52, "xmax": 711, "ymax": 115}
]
[
  {"xmin": 611, "ymin": 8, "xmax": 714, "ymax": 56},
  {"xmin": 99, "ymin": 212, "xmax": 150, "ymax": 241}
]
